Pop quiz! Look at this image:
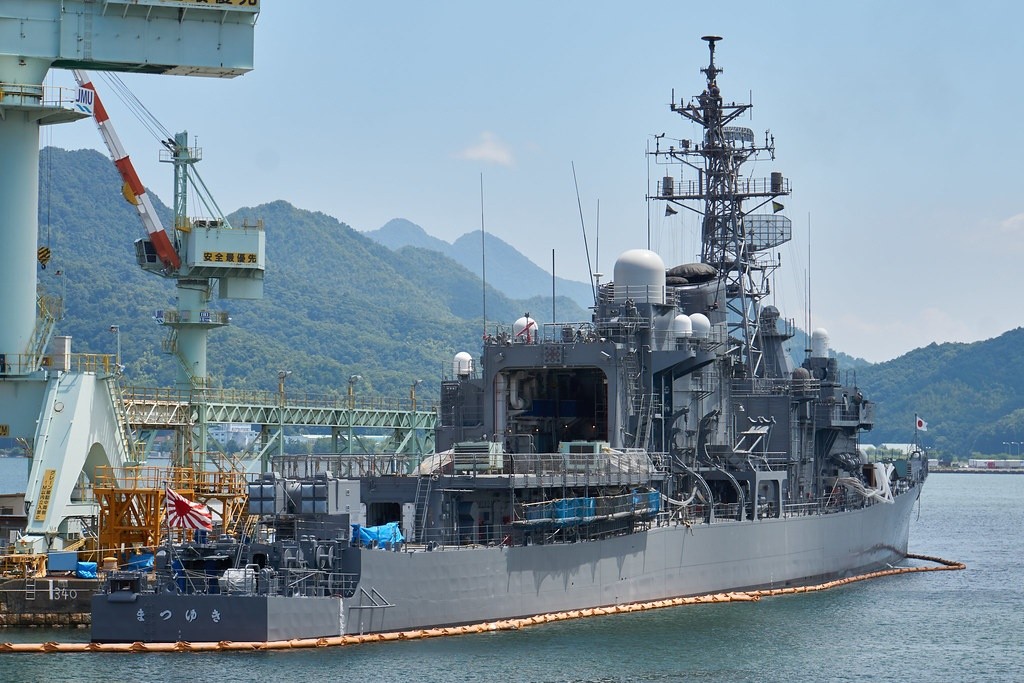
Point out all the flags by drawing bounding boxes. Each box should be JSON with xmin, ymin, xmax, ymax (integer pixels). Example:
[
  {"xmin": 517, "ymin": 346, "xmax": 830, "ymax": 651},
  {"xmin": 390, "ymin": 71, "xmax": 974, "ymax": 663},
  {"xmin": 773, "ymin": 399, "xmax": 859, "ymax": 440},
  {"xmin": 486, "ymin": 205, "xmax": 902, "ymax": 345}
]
[
  {"xmin": 166, "ymin": 487, "xmax": 212, "ymax": 529},
  {"xmin": 916, "ymin": 417, "xmax": 927, "ymax": 431}
]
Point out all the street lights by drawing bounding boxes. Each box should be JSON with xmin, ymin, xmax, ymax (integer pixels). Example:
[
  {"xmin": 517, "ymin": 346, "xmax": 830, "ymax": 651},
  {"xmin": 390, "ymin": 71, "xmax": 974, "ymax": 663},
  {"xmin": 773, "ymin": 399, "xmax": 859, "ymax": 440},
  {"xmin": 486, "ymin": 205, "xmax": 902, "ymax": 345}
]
[
  {"xmin": 1003, "ymin": 442, "xmax": 1010, "ymax": 458},
  {"xmin": 109, "ymin": 324, "xmax": 120, "ymax": 366},
  {"xmin": 1012, "ymin": 442, "xmax": 1020, "ymax": 458},
  {"xmin": 349, "ymin": 374, "xmax": 362, "ymax": 407},
  {"xmin": 278, "ymin": 369, "xmax": 294, "ymax": 402},
  {"xmin": 410, "ymin": 379, "xmax": 424, "ymax": 412}
]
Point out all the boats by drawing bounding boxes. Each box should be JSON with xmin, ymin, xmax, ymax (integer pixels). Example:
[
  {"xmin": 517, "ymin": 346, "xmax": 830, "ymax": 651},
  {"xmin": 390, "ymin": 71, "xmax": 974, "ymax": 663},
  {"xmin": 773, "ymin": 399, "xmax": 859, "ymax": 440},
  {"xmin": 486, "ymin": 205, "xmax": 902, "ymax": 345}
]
[{"xmin": 94, "ymin": 39, "xmax": 928, "ymax": 645}]
